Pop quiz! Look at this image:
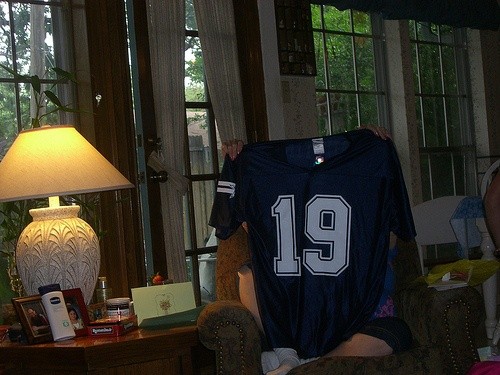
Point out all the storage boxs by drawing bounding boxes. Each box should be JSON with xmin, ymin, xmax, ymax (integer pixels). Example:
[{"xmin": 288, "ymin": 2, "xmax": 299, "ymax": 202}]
[{"xmin": 87, "ymin": 316, "xmax": 138, "ymax": 337}]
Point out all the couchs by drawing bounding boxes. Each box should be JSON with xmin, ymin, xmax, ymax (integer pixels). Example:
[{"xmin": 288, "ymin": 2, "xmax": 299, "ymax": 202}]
[{"xmin": 196, "ymin": 224, "xmax": 480, "ymax": 375}]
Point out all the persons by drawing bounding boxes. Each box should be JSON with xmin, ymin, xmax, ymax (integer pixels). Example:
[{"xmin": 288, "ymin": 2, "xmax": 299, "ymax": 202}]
[
  {"xmin": 221, "ymin": 125, "xmax": 410, "ymax": 375},
  {"xmin": 27, "ymin": 306, "xmax": 86, "ymax": 330},
  {"xmin": 482, "ymin": 169, "xmax": 500, "ymax": 257}
]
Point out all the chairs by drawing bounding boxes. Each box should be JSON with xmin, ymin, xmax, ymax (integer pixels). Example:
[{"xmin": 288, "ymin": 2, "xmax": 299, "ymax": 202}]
[
  {"xmin": 412, "ymin": 193, "xmax": 479, "ymax": 292},
  {"xmin": 474, "ymin": 160, "xmax": 500, "ymax": 343}
]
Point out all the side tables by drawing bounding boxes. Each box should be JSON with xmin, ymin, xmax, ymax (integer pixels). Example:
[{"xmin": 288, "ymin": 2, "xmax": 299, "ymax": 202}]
[{"xmin": 0, "ymin": 325, "xmax": 210, "ymax": 375}]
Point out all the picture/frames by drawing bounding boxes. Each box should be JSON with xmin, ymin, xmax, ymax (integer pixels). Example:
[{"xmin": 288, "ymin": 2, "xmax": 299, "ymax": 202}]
[
  {"xmin": 62, "ymin": 288, "xmax": 90, "ymax": 337},
  {"xmin": 12, "ymin": 294, "xmax": 54, "ymax": 344}
]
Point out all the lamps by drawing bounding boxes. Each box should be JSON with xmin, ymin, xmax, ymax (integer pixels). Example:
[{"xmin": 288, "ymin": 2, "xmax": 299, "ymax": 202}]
[{"xmin": 0, "ymin": 125, "xmax": 137, "ymax": 301}]
